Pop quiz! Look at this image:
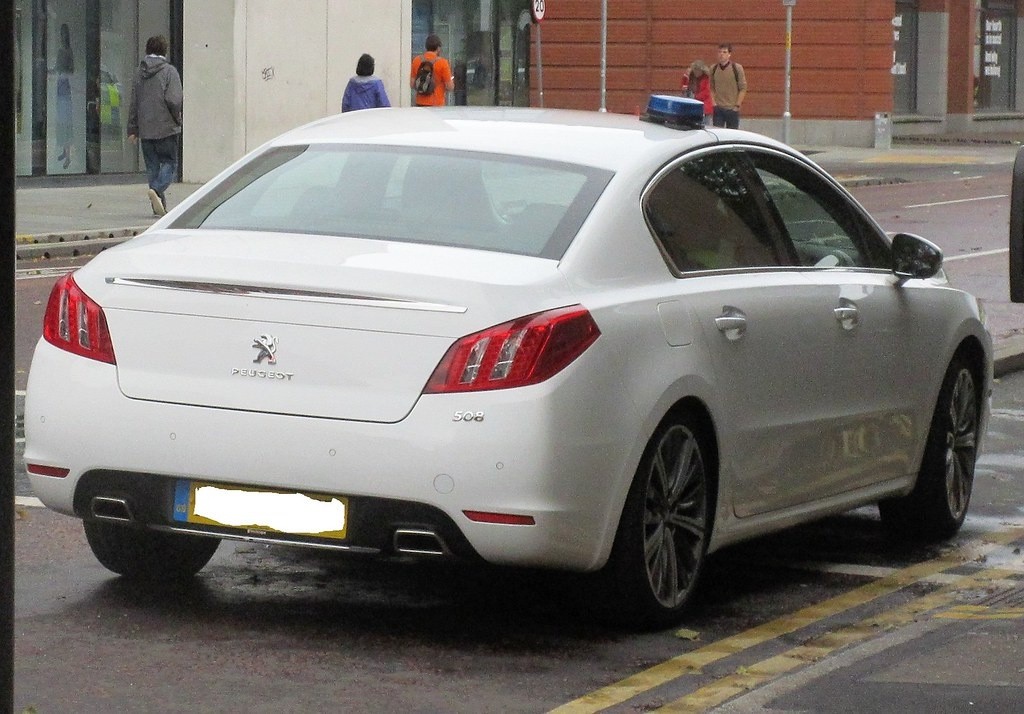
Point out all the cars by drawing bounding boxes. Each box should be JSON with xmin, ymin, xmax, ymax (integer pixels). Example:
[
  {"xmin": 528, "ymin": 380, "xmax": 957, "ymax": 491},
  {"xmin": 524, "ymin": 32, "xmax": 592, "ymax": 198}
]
[{"xmin": 20, "ymin": 104, "xmax": 994, "ymax": 623}]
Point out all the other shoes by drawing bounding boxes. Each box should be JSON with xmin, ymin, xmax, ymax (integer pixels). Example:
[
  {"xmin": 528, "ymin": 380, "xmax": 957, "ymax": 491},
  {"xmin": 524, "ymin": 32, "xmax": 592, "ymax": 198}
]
[{"xmin": 148, "ymin": 189, "xmax": 164, "ymax": 215}]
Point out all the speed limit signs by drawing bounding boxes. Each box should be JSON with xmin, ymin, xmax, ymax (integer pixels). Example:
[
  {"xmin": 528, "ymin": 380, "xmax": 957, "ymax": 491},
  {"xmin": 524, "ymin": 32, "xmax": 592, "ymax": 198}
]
[{"xmin": 531, "ymin": 0, "xmax": 546, "ymax": 23}]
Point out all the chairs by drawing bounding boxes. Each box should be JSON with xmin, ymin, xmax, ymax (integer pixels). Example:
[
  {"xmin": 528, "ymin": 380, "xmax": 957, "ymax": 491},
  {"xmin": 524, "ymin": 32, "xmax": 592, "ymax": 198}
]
[
  {"xmin": 647, "ymin": 169, "xmax": 779, "ymax": 270},
  {"xmin": 400, "ymin": 156, "xmax": 511, "ymax": 232}
]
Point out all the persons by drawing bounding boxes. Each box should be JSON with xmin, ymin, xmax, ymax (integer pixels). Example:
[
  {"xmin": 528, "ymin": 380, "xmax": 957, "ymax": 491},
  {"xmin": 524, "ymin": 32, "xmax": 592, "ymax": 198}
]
[
  {"xmin": 410, "ymin": 35, "xmax": 455, "ymax": 107},
  {"xmin": 681, "ymin": 59, "xmax": 714, "ymax": 126},
  {"xmin": 128, "ymin": 34, "xmax": 183, "ymax": 217},
  {"xmin": 341, "ymin": 54, "xmax": 391, "ymax": 113},
  {"xmin": 48, "ymin": 23, "xmax": 76, "ymax": 170},
  {"xmin": 708, "ymin": 42, "xmax": 747, "ymax": 130}
]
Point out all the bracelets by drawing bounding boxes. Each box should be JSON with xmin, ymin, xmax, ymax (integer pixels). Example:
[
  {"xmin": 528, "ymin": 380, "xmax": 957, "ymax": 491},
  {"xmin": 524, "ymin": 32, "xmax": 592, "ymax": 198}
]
[{"xmin": 734, "ymin": 105, "xmax": 740, "ymax": 108}]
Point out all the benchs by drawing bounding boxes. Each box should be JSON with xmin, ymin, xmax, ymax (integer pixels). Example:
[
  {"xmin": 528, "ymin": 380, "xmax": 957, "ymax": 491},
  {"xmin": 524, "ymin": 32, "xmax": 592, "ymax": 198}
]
[{"xmin": 286, "ymin": 187, "xmax": 569, "ymax": 251}]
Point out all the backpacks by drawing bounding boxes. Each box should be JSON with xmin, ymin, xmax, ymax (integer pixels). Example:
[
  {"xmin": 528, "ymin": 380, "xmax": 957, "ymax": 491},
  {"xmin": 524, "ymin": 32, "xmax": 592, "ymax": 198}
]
[{"xmin": 414, "ymin": 53, "xmax": 442, "ymax": 95}]
